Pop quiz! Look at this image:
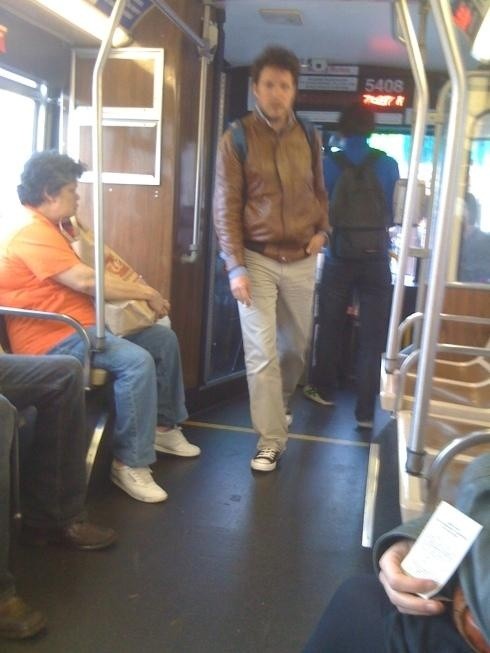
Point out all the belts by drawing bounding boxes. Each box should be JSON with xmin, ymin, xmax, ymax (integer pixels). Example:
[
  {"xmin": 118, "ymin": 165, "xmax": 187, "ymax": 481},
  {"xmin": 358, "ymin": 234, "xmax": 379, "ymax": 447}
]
[{"xmin": 451, "ymin": 580, "xmax": 490, "ymax": 652}]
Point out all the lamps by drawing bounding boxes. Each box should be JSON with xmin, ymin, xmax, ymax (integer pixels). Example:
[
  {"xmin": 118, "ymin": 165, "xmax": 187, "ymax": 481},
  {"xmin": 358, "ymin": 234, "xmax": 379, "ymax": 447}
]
[{"xmin": 32, "ymin": 0, "xmax": 136, "ymax": 49}]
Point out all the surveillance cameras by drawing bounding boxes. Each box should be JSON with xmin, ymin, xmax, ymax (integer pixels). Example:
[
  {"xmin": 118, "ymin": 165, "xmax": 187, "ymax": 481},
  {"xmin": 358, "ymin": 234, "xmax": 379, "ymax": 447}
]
[{"xmin": 311, "ymin": 59, "xmax": 328, "ymax": 73}]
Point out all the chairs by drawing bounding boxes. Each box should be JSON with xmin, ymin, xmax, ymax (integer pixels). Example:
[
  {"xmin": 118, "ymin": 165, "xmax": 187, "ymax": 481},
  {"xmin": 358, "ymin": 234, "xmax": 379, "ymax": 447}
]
[
  {"xmin": 0, "ymin": 305, "xmax": 173, "ymax": 535},
  {"xmin": 379, "ymin": 310, "xmax": 490, "ymax": 526}
]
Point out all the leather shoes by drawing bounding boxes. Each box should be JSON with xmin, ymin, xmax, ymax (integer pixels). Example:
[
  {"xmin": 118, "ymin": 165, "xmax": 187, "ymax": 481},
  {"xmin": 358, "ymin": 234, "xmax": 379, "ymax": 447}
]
[
  {"xmin": 21, "ymin": 518, "xmax": 119, "ymax": 549},
  {"xmin": 1, "ymin": 595, "xmax": 48, "ymax": 640}
]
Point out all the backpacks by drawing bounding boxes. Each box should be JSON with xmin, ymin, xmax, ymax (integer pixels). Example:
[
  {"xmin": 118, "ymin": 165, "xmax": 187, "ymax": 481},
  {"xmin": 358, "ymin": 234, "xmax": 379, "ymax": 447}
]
[{"xmin": 328, "ymin": 148, "xmax": 394, "ymax": 262}]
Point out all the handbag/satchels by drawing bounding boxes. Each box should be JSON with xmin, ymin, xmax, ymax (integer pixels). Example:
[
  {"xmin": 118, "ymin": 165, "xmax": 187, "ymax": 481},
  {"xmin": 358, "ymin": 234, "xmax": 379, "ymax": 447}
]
[{"xmin": 58, "ymin": 214, "xmax": 164, "ymax": 338}]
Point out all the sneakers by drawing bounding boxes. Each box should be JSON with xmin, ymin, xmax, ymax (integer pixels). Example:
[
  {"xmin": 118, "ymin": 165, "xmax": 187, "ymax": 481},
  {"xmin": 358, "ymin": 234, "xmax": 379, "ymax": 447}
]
[
  {"xmin": 303, "ymin": 384, "xmax": 335, "ymax": 406},
  {"xmin": 284, "ymin": 399, "xmax": 293, "ymax": 426},
  {"xmin": 109, "ymin": 456, "xmax": 168, "ymax": 503},
  {"xmin": 358, "ymin": 420, "xmax": 374, "ymax": 428},
  {"xmin": 250, "ymin": 449, "xmax": 280, "ymax": 471},
  {"xmin": 153, "ymin": 426, "xmax": 201, "ymax": 457}
]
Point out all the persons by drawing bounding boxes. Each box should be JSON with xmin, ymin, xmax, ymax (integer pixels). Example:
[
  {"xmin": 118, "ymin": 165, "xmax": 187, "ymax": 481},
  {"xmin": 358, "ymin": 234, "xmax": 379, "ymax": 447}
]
[
  {"xmin": 459, "ymin": 193, "xmax": 490, "ymax": 282},
  {"xmin": 0, "ymin": 353, "xmax": 117, "ymax": 640},
  {"xmin": 212, "ymin": 44, "xmax": 330, "ymax": 472},
  {"xmin": 0, "ymin": 150, "xmax": 200, "ymax": 502},
  {"xmin": 304, "ymin": 103, "xmax": 401, "ymax": 430},
  {"xmin": 310, "ymin": 450, "xmax": 488, "ymax": 652}
]
